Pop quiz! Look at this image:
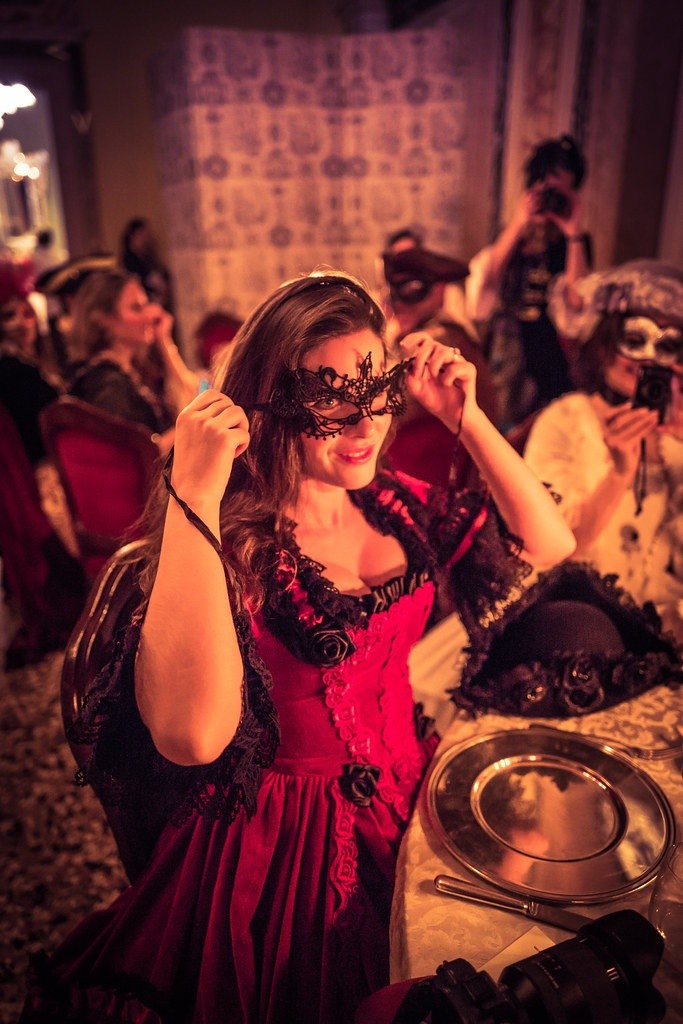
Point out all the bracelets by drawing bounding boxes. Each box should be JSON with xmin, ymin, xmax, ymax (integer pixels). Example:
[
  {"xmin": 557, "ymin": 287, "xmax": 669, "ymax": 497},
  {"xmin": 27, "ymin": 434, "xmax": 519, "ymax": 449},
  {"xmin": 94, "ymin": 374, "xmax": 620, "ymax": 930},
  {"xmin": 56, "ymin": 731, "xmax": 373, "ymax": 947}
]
[{"xmin": 564, "ymin": 229, "xmax": 592, "ymax": 244}]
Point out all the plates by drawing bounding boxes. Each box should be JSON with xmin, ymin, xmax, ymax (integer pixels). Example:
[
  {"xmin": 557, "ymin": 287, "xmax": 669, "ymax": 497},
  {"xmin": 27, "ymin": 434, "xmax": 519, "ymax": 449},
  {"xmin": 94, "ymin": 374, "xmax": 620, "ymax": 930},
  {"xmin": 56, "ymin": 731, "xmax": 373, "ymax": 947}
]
[{"xmin": 425, "ymin": 727, "xmax": 677, "ymax": 905}]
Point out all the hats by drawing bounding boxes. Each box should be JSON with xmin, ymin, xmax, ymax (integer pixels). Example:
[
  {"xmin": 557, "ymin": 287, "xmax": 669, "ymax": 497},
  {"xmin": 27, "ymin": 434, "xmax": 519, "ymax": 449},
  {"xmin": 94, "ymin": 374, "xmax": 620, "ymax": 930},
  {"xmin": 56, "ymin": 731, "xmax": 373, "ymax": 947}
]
[
  {"xmin": 380, "ymin": 248, "xmax": 471, "ymax": 283},
  {"xmin": 593, "ymin": 258, "xmax": 683, "ymax": 331},
  {"xmin": 445, "ymin": 559, "xmax": 683, "ymax": 720},
  {"xmin": 34, "ymin": 251, "xmax": 117, "ymax": 298}
]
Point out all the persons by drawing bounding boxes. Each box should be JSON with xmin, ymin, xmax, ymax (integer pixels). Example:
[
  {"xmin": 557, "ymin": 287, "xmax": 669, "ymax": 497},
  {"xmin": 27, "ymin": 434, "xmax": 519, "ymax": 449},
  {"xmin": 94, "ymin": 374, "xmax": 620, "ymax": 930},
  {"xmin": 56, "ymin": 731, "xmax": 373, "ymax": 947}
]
[
  {"xmin": 521, "ymin": 258, "xmax": 682, "ymax": 649},
  {"xmin": 376, "ymin": 226, "xmax": 501, "ymax": 445},
  {"xmin": 0, "ymin": 217, "xmax": 214, "ymax": 1023},
  {"xmin": 466, "ymin": 128, "xmax": 634, "ymax": 456},
  {"xmin": 42, "ymin": 265, "xmax": 578, "ymax": 1024}
]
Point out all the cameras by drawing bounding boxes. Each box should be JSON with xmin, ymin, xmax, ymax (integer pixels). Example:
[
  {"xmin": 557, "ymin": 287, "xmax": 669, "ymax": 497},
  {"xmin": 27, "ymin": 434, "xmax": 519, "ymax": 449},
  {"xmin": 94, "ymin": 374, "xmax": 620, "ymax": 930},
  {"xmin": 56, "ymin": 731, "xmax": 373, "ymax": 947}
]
[
  {"xmin": 536, "ymin": 185, "xmax": 567, "ymax": 215},
  {"xmin": 389, "ymin": 909, "xmax": 666, "ymax": 1024},
  {"xmin": 631, "ymin": 364, "xmax": 674, "ymax": 426}
]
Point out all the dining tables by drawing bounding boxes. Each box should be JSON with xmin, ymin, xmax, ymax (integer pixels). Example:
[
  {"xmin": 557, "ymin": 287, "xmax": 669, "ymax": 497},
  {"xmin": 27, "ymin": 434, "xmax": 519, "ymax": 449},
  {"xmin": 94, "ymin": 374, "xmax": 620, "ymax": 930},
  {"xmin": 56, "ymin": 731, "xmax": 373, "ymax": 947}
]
[{"xmin": 387, "ymin": 684, "xmax": 683, "ymax": 1024}]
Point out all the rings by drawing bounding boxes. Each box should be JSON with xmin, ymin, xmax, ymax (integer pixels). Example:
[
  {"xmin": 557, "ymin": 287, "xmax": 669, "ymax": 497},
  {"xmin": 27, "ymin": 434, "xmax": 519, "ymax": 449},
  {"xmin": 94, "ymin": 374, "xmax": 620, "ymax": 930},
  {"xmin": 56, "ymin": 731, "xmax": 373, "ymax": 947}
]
[{"xmin": 452, "ymin": 347, "xmax": 462, "ymax": 365}]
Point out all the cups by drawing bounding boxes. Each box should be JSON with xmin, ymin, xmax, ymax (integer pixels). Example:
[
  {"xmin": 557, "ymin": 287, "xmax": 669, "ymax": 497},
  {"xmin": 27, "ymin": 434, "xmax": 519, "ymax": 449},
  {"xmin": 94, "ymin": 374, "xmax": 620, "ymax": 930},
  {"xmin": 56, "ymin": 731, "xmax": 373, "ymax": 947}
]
[{"xmin": 648, "ymin": 842, "xmax": 683, "ymax": 970}]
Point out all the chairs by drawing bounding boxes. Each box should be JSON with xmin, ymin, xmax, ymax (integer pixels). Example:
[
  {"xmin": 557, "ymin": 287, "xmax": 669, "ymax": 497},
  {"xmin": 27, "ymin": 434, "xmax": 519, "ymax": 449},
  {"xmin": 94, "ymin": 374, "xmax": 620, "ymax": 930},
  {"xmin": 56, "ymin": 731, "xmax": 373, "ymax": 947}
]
[
  {"xmin": 195, "ymin": 309, "xmax": 244, "ymax": 370},
  {"xmin": 41, "ymin": 396, "xmax": 163, "ymax": 584},
  {"xmin": 1, "ymin": 409, "xmax": 77, "ymax": 660},
  {"xmin": 59, "ymin": 539, "xmax": 199, "ymax": 885}
]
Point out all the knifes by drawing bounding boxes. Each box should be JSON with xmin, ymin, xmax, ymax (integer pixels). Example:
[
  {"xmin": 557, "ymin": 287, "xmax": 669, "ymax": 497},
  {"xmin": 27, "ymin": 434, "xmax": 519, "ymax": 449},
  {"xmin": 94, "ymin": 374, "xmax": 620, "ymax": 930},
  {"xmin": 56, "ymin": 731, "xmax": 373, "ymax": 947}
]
[{"xmin": 433, "ymin": 874, "xmax": 595, "ymax": 934}]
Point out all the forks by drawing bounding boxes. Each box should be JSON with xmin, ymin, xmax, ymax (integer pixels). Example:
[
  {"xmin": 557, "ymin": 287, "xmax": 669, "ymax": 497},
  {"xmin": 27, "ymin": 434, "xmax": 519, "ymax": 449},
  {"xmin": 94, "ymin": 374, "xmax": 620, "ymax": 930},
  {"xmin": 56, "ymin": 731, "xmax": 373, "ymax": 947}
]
[{"xmin": 530, "ymin": 722, "xmax": 683, "ymax": 763}]
